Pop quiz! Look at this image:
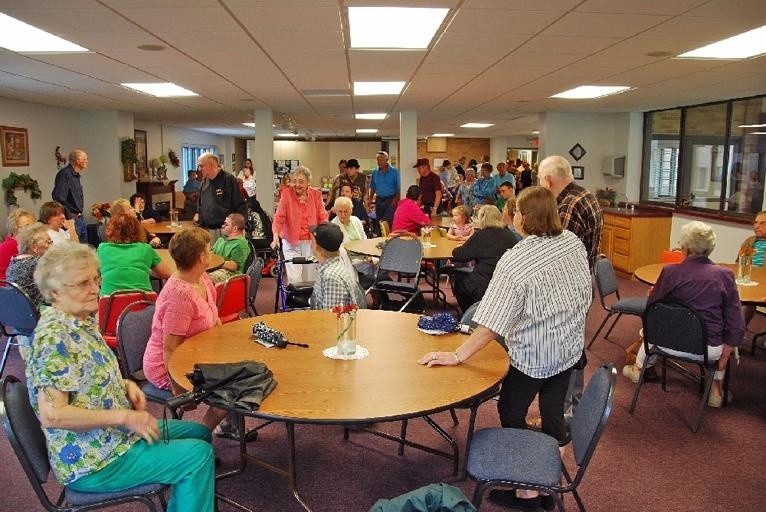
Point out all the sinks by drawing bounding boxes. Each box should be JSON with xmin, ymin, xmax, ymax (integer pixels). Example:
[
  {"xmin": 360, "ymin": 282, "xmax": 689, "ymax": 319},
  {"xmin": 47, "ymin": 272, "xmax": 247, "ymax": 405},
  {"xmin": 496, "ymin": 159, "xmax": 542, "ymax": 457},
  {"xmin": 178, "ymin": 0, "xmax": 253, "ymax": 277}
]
[{"xmin": 605, "ymin": 205, "xmax": 639, "ymax": 216}]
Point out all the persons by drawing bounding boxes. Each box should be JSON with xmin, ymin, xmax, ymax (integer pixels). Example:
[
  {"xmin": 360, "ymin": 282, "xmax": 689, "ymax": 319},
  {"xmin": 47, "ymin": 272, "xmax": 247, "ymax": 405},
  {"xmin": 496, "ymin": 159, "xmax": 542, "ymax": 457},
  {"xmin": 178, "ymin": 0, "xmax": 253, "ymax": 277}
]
[
  {"xmin": 3, "ymin": 150, "xmax": 602, "ymax": 511},
  {"xmin": 623, "ymin": 220, "xmax": 747, "ymax": 408},
  {"xmin": 728, "ymin": 161, "xmax": 764, "ymax": 213},
  {"xmin": 735, "ymin": 210, "xmax": 766, "ymax": 324}
]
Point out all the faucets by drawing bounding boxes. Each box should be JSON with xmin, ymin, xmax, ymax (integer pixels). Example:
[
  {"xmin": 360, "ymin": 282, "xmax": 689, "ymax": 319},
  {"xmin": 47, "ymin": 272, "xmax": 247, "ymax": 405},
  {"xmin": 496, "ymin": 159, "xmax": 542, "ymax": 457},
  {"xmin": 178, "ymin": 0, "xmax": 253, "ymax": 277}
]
[{"xmin": 620, "ymin": 191, "xmax": 633, "ymax": 210}]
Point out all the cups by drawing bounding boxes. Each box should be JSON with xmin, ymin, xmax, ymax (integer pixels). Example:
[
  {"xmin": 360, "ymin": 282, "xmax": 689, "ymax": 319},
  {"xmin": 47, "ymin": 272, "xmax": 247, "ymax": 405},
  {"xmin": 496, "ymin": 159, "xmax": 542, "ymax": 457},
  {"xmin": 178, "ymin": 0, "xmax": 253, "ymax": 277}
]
[{"xmin": 170, "ymin": 211, "xmax": 178, "ymax": 227}]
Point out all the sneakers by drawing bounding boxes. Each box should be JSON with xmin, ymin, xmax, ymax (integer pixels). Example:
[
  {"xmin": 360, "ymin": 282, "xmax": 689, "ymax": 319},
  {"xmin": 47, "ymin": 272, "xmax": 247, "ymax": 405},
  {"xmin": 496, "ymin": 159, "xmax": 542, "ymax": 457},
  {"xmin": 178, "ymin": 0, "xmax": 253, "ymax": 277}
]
[
  {"xmin": 488, "ymin": 489, "xmax": 555, "ymax": 511},
  {"xmin": 707, "ymin": 390, "xmax": 734, "ymax": 408},
  {"xmin": 621, "ymin": 364, "xmax": 642, "ymax": 383},
  {"xmin": 216, "ymin": 423, "xmax": 256, "ymax": 443}
]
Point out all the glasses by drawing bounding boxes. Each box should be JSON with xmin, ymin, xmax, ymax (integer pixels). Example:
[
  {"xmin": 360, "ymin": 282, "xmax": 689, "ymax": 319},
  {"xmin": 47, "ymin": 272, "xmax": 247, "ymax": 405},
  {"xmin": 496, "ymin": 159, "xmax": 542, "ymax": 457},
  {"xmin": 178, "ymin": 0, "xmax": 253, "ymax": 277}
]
[
  {"xmin": 36, "ymin": 238, "xmax": 54, "ymax": 246},
  {"xmin": 63, "ymin": 276, "xmax": 103, "ymax": 290},
  {"xmin": 512, "ymin": 208, "xmax": 523, "ymax": 216}
]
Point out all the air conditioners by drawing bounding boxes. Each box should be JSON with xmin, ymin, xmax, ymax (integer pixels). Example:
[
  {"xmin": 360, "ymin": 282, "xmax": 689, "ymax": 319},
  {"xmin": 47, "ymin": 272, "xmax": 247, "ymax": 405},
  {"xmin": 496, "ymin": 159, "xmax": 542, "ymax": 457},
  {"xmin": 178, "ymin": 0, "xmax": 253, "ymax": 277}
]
[{"xmin": 602, "ymin": 154, "xmax": 625, "ymax": 178}]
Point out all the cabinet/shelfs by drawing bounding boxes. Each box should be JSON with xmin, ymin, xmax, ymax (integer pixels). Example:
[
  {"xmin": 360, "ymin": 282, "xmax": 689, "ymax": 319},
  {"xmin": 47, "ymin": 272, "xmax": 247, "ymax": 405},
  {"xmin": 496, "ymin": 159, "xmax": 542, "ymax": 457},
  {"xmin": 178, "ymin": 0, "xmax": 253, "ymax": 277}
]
[{"xmin": 137, "ymin": 178, "xmax": 176, "ymax": 218}]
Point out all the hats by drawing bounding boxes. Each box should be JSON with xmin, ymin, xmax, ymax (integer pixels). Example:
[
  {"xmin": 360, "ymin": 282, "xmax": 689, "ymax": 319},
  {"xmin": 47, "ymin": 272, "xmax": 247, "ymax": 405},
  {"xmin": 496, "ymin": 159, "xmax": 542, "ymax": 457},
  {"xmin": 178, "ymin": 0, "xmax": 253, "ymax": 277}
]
[
  {"xmin": 414, "ymin": 157, "xmax": 430, "ymax": 170},
  {"xmin": 343, "ymin": 159, "xmax": 360, "ymax": 170},
  {"xmin": 306, "ymin": 222, "xmax": 343, "ymax": 249}
]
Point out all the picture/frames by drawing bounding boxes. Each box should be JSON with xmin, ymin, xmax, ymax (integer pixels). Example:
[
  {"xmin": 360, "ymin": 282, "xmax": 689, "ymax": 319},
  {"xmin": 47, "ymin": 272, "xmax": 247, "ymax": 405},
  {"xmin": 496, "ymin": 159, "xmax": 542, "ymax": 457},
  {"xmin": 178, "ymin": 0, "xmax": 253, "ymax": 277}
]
[
  {"xmin": 571, "ymin": 166, "xmax": 584, "ymax": 180},
  {"xmin": 569, "ymin": 143, "xmax": 587, "ymax": 162},
  {"xmin": 134, "ymin": 129, "xmax": 147, "ymax": 174}
]
